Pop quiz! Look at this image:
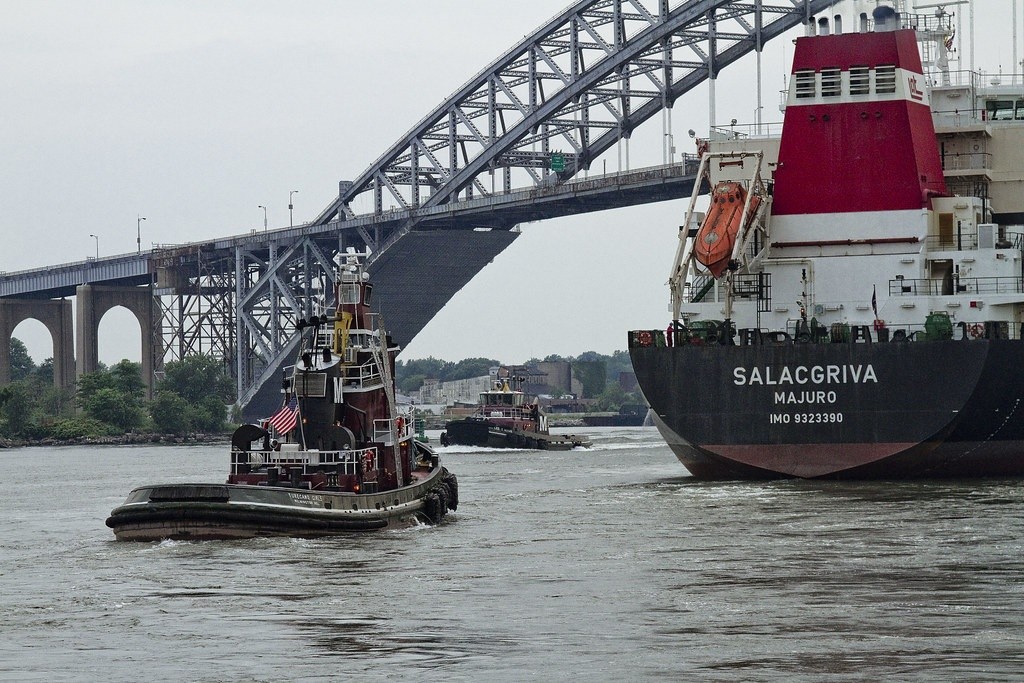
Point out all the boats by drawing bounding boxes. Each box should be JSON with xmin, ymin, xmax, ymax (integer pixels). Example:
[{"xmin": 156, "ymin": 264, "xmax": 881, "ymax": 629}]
[
  {"xmin": 582, "ymin": 403, "xmax": 654, "ymax": 427},
  {"xmin": 625, "ymin": 0, "xmax": 1023, "ymax": 480},
  {"xmin": 691, "ymin": 180, "xmax": 762, "ymax": 280},
  {"xmin": 440, "ymin": 376, "xmax": 594, "ymax": 452},
  {"xmin": 104, "ymin": 250, "xmax": 459, "ymax": 547}
]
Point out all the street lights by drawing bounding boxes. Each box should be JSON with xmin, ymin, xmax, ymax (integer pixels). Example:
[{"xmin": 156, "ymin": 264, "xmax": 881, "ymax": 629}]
[{"xmin": 88, "ymin": 190, "xmax": 301, "ymax": 262}]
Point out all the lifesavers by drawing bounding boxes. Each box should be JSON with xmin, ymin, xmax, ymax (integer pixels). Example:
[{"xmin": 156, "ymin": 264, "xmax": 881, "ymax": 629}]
[
  {"xmin": 638, "ymin": 332, "xmax": 653, "ymax": 345},
  {"xmin": 368, "ymin": 452, "xmax": 375, "ymax": 469}
]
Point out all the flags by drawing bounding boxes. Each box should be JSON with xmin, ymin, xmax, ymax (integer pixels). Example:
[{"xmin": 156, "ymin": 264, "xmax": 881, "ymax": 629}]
[
  {"xmin": 269, "ymin": 397, "xmax": 301, "ymax": 436},
  {"xmin": 945, "ymin": 34, "xmax": 956, "ymax": 51}
]
